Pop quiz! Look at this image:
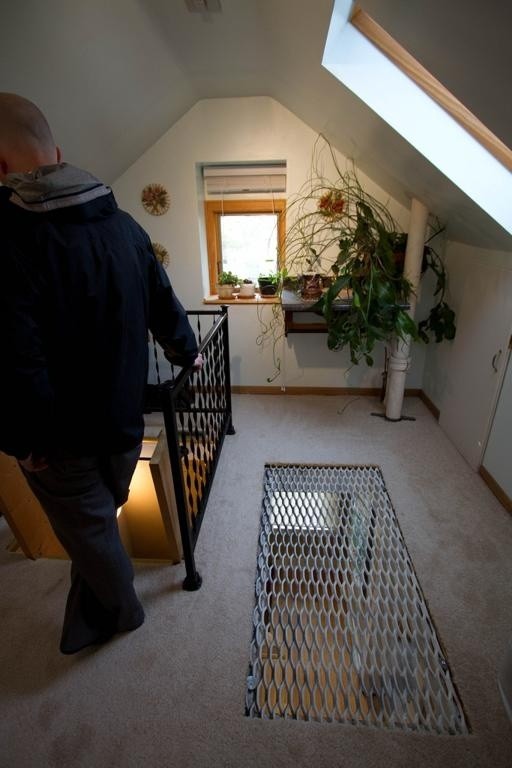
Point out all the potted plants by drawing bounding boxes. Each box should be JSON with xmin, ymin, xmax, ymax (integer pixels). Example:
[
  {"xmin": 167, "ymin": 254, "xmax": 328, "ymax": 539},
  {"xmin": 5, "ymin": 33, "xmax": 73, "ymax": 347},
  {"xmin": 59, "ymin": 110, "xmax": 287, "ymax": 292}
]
[{"xmin": 214, "ymin": 270, "xmax": 283, "ymax": 299}]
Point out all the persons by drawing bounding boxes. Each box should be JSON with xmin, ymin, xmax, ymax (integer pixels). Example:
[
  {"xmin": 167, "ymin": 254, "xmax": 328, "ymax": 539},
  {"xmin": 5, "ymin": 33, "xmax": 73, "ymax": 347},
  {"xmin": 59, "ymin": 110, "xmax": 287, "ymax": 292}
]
[{"xmin": 0, "ymin": 83, "xmax": 211, "ymax": 659}]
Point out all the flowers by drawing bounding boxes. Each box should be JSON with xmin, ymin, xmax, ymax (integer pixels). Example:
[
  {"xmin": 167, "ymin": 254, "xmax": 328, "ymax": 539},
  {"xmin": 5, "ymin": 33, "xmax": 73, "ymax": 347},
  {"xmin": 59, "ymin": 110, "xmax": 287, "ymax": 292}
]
[{"xmin": 259, "ymin": 141, "xmax": 456, "ymax": 386}]
